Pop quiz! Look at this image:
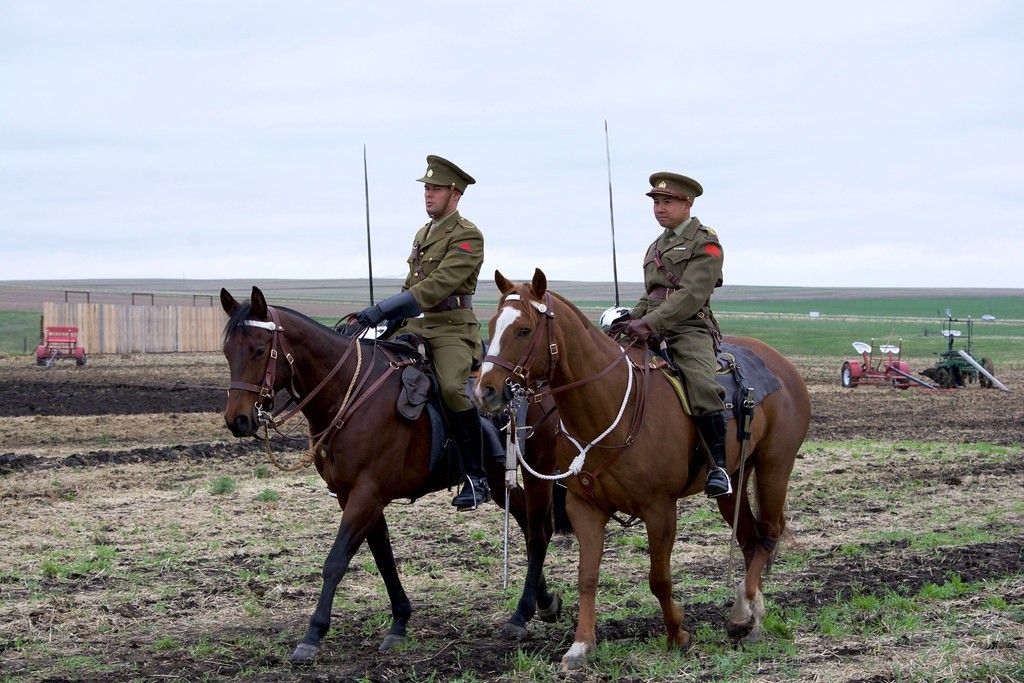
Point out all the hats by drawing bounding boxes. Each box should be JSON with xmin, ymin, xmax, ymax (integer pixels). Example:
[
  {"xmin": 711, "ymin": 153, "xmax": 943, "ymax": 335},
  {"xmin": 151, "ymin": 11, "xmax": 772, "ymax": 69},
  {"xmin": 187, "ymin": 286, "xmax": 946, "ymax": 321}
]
[
  {"xmin": 646, "ymin": 172, "xmax": 703, "ymax": 206},
  {"xmin": 416, "ymin": 155, "xmax": 476, "ymax": 195}
]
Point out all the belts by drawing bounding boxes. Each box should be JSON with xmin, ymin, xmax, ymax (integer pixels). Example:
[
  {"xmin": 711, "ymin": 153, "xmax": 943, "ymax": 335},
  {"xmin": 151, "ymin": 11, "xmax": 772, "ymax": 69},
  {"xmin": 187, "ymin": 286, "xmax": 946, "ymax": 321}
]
[
  {"xmin": 423, "ymin": 295, "xmax": 472, "ymax": 312},
  {"xmin": 648, "ymin": 286, "xmax": 676, "ymax": 300}
]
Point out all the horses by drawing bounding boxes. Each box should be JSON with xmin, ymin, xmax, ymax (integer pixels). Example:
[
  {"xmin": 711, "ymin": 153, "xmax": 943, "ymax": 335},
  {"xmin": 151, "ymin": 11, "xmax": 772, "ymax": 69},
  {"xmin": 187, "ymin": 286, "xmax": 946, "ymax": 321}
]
[
  {"xmin": 220, "ymin": 285, "xmax": 573, "ymax": 668},
  {"xmin": 472, "ymin": 268, "xmax": 810, "ymax": 669}
]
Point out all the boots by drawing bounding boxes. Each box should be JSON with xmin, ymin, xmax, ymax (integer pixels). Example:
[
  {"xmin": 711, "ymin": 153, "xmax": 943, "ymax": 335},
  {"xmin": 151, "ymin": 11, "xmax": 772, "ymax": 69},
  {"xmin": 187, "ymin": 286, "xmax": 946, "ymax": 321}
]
[
  {"xmin": 449, "ymin": 406, "xmax": 491, "ymax": 508},
  {"xmin": 697, "ymin": 415, "xmax": 733, "ymax": 498}
]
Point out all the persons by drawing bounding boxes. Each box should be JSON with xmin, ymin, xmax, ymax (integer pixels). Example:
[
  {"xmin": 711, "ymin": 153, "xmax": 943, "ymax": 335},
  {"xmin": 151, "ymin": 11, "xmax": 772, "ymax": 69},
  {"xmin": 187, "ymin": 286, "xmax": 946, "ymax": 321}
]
[
  {"xmin": 623, "ymin": 172, "xmax": 733, "ymax": 496},
  {"xmin": 357, "ymin": 155, "xmax": 492, "ymax": 508}
]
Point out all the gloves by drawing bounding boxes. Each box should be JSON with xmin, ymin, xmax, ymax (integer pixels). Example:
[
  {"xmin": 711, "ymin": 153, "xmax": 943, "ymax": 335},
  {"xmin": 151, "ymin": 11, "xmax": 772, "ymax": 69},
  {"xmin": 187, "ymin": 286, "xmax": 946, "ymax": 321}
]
[
  {"xmin": 356, "ymin": 290, "xmax": 420, "ymax": 328},
  {"xmin": 624, "ymin": 319, "xmax": 652, "ymax": 343}
]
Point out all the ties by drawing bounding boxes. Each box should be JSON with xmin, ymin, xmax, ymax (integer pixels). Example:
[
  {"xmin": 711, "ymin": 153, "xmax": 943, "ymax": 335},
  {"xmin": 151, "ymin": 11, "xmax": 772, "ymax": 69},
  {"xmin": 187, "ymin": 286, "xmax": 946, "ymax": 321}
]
[{"xmin": 426, "ymin": 222, "xmax": 437, "ymax": 241}]
